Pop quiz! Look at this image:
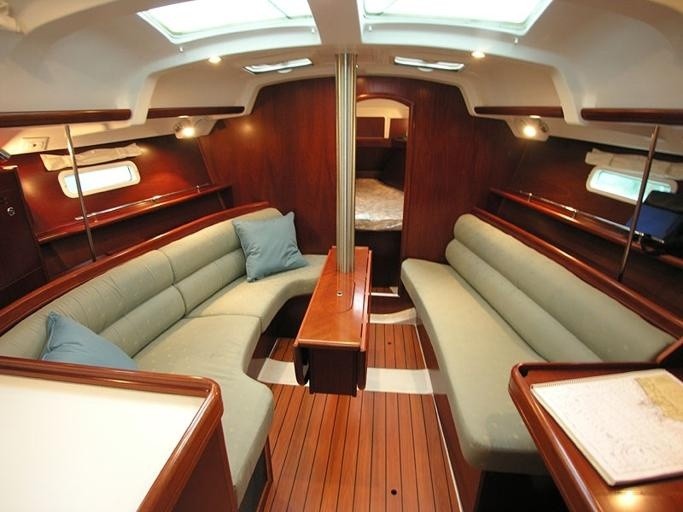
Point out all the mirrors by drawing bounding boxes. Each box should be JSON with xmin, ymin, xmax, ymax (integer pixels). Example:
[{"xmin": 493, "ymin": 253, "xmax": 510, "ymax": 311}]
[{"xmin": 354, "ymin": 91, "xmax": 413, "ymax": 288}]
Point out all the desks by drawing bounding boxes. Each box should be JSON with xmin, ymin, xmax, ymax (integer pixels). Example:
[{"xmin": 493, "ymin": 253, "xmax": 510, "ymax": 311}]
[{"xmin": 503, "ymin": 358, "xmax": 683, "ymax": 510}]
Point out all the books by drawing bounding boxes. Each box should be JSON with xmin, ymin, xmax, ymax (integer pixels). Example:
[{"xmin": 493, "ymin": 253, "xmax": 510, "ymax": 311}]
[{"xmin": 530, "ymin": 368, "xmax": 683, "ymax": 488}]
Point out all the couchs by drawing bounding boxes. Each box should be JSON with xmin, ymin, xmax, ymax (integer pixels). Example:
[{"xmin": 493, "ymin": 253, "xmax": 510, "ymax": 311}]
[
  {"xmin": 0, "ymin": 198, "xmax": 328, "ymax": 512},
  {"xmin": 397, "ymin": 206, "xmax": 682, "ymax": 511}
]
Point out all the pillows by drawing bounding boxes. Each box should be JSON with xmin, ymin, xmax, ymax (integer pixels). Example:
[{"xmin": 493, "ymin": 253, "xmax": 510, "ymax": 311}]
[
  {"xmin": 228, "ymin": 210, "xmax": 312, "ymax": 282},
  {"xmin": 34, "ymin": 310, "xmax": 138, "ymax": 373}
]
